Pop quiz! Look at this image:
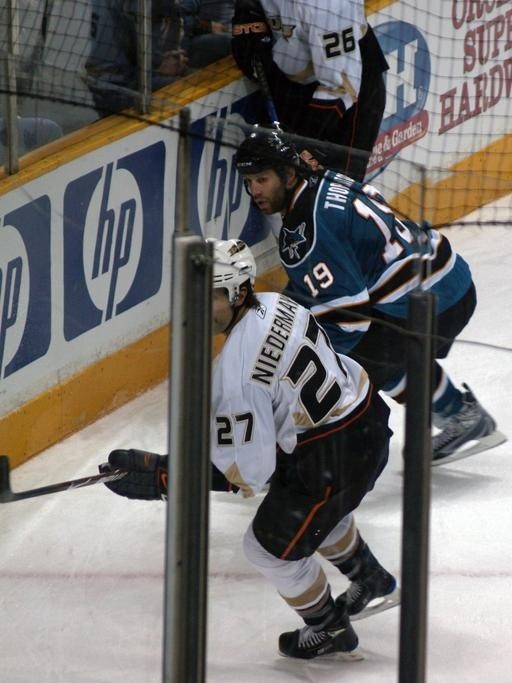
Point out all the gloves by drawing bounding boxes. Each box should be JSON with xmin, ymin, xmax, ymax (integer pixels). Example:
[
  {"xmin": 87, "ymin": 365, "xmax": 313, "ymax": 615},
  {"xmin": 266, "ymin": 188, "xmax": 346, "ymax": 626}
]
[
  {"xmin": 231, "ymin": 0, "xmax": 279, "ymax": 83},
  {"xmin": 294, "ymin": 97, "xmax": 346, "ymax": 165},
  {"xmin": 99, "ymin": 449, "xmax": 168, "ymax": 500}
]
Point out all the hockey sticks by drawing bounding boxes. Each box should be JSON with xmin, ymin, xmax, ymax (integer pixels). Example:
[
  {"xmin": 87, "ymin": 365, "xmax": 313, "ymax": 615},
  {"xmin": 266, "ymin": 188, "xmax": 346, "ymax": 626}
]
[{"xmin": 0, "ymin": 455, "xmax": 131, "ymax": 501}]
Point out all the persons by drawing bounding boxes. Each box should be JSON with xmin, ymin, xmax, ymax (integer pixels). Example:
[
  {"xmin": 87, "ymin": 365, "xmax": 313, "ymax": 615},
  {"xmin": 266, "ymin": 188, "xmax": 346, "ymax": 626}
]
[
  {"xmin": 176, "ymin": 0, "xmax": 236, "ymax": 71},
  {"xmin": 231, "ymin": 133, "xmax": 499, "ymax": 466},
  {"xmin": 228, "ymin": 1, "xmax": 393, "ymax": 184},
  {"xmin": 96, "ymin": 236, "xmax": 397, "ymax": 663},
  {"xmin": 84, "ymin": 0, "xmax": 203, "ymax": 123}
]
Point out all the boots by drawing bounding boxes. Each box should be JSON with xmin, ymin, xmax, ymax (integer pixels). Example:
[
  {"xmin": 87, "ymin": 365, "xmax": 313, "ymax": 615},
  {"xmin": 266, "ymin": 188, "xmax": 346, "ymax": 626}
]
[
  {"xmin": 431, "ymin": 383, "xmax": 495, "ymax": 460},
  {"xmin": 334, "ymin": 541, "xmax": 396, "ymax": 616},
  {"xmin": 278, "ymin": 600, "xmax": 358, "ymax": 659}
]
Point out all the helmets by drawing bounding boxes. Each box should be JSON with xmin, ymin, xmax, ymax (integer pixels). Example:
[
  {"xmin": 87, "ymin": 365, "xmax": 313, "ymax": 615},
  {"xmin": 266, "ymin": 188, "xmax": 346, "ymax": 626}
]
[
  {"xmin": 204, "ymin": 236, "xmax": 257, "ymax": 308},
  {"xmin": 235, "ymin": 132, "xmax": 300, "ymax": 174}
]
